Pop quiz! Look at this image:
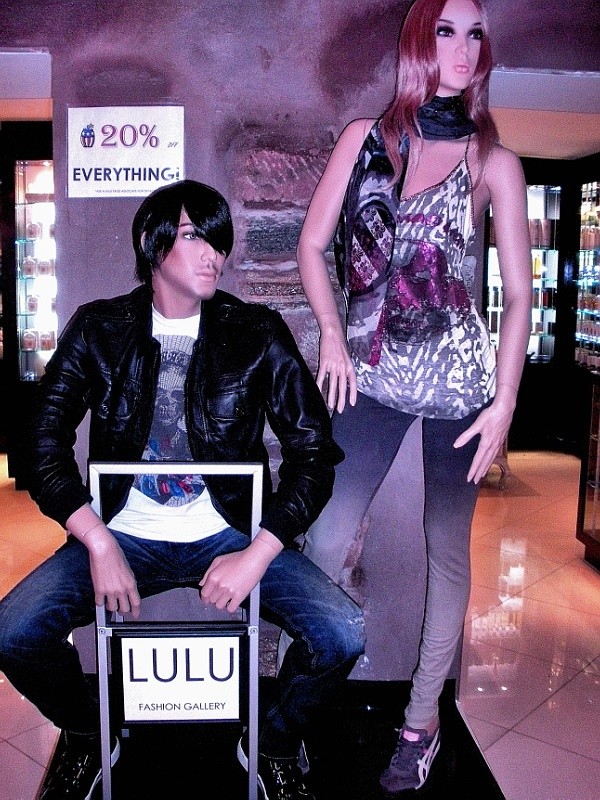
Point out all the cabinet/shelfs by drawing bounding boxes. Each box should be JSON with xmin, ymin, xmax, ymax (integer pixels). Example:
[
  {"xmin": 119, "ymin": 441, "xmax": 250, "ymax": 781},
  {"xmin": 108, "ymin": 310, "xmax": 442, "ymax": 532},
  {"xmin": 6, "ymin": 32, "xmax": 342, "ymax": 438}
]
[
  {"xmin": 481, "ymin": 151, "xmax": 600, "ymax": 462},
  {"xmin": 0, "ymin": 120, "xmax": 58, "ymax": 490}
]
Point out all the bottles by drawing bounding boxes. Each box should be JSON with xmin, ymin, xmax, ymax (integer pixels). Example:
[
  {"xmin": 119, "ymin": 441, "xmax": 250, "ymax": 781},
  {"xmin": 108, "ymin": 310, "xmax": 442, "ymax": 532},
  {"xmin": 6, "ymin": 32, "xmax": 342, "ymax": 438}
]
[{"xmin": 21, "ymin": 219, "xmax": 58, "ymax": 351}]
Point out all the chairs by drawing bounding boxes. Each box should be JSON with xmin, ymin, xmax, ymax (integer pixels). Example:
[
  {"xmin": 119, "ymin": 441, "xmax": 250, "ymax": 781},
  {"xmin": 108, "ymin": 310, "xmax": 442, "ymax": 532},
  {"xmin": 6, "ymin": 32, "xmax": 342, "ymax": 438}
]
[{"xmin": 87, "ymin": 460, "xmax": 264, "ymax": 800}]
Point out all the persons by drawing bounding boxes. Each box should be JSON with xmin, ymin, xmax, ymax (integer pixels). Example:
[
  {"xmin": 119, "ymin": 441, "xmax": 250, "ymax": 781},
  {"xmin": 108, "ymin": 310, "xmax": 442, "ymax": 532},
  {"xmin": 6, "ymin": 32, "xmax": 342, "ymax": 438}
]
[
  {"xmin": 277, "ymin": 0, "xmax": 533, "ymax": 800},
  {"xmin": 0, "ymin": 177, "xmax": 367, "ymax": 800}
]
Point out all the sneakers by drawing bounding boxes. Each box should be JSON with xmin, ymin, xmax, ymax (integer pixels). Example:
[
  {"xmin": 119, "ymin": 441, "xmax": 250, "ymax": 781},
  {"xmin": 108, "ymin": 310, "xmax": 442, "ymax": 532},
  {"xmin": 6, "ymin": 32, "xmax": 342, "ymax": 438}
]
[
  {"xmin": 237, "ymin": 728, "xmax": 315, "ymax": 800},
  {"xmin": 40, "ymin": 727, "xmax": 121, "ymax": 800},
  {"xmin": 379, "ymin": 721, "xmax": 442, "ymax": 794}
]
